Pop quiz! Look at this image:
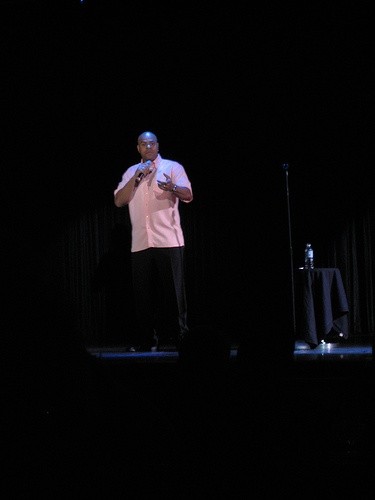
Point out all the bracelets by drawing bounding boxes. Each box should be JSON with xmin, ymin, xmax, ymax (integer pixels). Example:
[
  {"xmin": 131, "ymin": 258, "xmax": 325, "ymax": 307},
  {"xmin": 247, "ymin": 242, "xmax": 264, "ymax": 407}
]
[{"xmin": 169, "ymin": 184, "xmax": 177, "ymax": 192}]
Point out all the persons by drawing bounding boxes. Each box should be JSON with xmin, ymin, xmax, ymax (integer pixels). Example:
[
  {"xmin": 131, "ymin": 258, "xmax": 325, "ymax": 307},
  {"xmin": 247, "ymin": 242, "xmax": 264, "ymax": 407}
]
[{"xmin": 114, "ymin": 132, "xmax": 194, "ymax": 352}]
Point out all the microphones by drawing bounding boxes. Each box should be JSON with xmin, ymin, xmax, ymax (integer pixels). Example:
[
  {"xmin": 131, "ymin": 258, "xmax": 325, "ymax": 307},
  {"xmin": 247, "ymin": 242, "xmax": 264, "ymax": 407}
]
[{"xmin": 136, "ymin": 161, "xmax": 151, "ymax": 183}]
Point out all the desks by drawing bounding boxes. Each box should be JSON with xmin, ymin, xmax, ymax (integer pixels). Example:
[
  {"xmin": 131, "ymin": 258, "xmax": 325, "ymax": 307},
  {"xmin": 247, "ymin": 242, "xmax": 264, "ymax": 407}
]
[{"xmin": 294, "ymin": 268, "xmax": 350, "ymax": 349}]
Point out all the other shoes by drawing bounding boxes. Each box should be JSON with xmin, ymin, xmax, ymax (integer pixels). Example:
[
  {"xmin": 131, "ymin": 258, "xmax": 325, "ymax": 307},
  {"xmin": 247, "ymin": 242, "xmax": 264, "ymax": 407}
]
[{"xmin": 128, "ymin": 342, "xmax": 158, "ymax": 353}]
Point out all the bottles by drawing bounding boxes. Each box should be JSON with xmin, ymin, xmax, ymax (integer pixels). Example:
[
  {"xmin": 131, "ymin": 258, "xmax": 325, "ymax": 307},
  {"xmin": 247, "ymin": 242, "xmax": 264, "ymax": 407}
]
[{"xmin": 305, "ymin": 244, "xmax": 314, "ymax": 269}]
